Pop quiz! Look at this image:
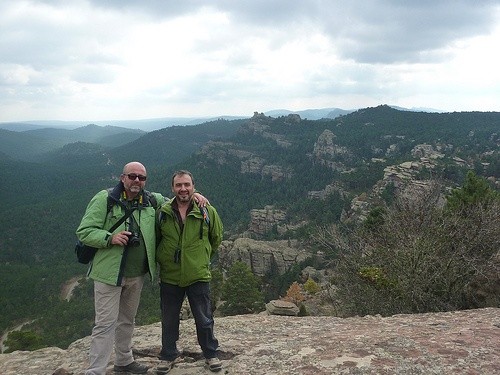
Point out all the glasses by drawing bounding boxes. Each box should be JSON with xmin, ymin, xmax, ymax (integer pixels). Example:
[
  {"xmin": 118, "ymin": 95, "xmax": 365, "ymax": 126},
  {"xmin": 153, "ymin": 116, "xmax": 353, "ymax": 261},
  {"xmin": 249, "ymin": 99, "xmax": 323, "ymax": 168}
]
[{"xmin": 124, "ymin": 174, "xmax": 147, "ymax": 181}]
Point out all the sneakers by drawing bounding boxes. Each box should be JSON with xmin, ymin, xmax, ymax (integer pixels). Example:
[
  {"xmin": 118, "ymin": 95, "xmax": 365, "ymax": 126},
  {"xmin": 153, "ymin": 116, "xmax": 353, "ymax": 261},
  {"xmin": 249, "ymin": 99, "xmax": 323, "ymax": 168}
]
[
  {"xmin": 157, "ymin": 359, "xmax": 176, "ymax": 373},
  {"xmin": 205, "ymin": 358, "xmax": 223, "ymax": 371},
  {"xmin": 114, "ymin": 362, "xmax": 147, "ymax": 373}
]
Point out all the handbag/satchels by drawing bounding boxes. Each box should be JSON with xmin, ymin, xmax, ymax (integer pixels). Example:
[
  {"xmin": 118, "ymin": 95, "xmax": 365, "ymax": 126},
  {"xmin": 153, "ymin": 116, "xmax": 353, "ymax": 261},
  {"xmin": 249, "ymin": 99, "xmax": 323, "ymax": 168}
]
[{"xmin": 76, "ymin": 241, "xmax": 98, "ymax": 264}]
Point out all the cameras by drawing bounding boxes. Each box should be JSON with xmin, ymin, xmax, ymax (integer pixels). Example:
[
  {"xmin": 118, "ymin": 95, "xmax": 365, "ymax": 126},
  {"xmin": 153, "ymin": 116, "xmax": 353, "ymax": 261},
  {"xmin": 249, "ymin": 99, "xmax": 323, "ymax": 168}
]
[{"xmin": 125, "ymin": 229, "xmax": 141, "ymax": 247}]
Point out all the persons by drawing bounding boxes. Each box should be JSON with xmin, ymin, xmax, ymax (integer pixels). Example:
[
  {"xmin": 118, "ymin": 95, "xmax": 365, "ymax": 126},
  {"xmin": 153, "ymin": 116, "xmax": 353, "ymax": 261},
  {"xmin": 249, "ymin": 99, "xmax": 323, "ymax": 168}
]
[
  {"xmin": 75, "ymin": 162, "xmax": 211, "ymax": 375},
  {"xmin": 154, "ymin": 169, "xmax": 224, "ymax": 375}
]
